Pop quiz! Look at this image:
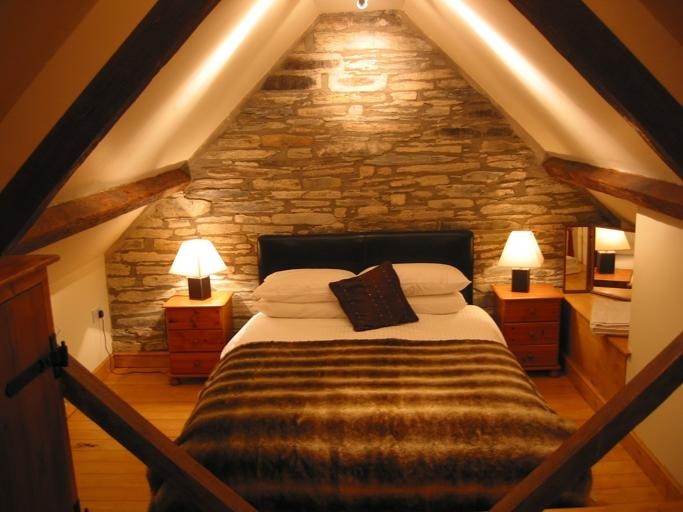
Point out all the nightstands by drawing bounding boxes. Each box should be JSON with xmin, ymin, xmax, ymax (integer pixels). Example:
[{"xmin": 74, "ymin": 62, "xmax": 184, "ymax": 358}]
[
  {"xmin": 161, "ymin": 291, "xmax": 233, "ymax": 386},
  {"xmin": 491, "ymin": 282, "xmax": 565, "ymax": 378}
]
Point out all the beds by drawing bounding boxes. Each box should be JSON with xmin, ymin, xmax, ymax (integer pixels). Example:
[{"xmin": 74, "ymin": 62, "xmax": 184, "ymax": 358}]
[{"xmin": 150, "ymin": 229, "xmax": 596, "ymax": 512}]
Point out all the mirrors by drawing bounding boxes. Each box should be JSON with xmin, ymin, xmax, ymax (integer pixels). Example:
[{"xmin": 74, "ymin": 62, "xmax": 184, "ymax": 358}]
[{"xmin": 563, "ymin": 223, "xmax": 636, "ymax": 304}]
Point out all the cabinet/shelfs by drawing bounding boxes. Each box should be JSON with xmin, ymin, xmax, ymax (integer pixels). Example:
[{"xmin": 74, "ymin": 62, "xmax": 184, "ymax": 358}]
[{"xmin": 0, "ymin": 254, "xmax": 84, "ymax": 512}]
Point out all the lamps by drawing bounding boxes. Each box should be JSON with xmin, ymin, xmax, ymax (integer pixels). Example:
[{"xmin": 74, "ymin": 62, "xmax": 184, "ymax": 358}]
[
  {"xmin": 168, "ymin": 239, "xmax": 227, "ymax": 300},
  {"xmin": 496, "ymin": 231, "xmax": 545, "ymax": 294}
]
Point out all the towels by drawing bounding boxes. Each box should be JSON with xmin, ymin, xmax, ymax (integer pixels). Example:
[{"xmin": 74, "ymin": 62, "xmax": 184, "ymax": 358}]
[{"xmin": 590, "ymin": 298, "xmax": 630, "ymax": 337}]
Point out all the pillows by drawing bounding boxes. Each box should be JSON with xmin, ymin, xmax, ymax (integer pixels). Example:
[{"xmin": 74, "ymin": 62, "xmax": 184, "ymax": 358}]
[{"xmin": 249, "ymin": 259, "xmax": 472, "ymax": 333}]
